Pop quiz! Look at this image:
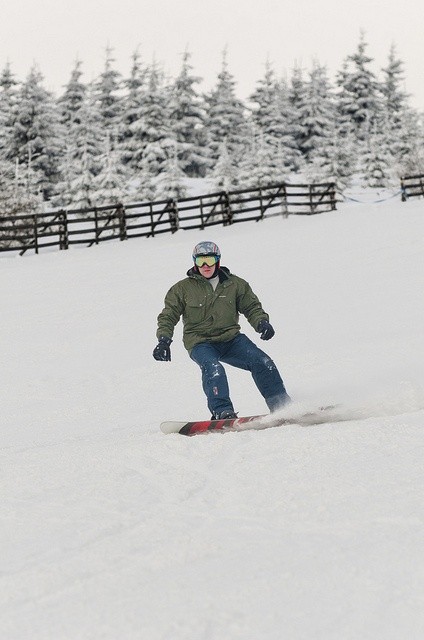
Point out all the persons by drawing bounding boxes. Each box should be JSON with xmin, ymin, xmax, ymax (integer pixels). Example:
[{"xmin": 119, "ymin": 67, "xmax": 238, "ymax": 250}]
[{"xmin": 153, "ymin": 241, "xmax": 295, "ymax": 420}]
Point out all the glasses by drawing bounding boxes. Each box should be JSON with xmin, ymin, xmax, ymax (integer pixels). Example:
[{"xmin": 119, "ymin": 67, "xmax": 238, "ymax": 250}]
[{"xmin": 193, "ymin": 255, "xmax": 220, "ymax": 268}]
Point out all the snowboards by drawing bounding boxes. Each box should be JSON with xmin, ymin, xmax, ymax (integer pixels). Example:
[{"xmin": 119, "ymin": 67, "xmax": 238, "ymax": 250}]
[{"xmin": 159, "ymin": 404, "xmax": 334, "ymax": 436}]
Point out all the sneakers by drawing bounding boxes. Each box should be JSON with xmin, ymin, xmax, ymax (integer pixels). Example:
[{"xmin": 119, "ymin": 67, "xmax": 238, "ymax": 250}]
[{"xmin": 211, "ymin": 410, "xmax": 239, "ymax": 419}]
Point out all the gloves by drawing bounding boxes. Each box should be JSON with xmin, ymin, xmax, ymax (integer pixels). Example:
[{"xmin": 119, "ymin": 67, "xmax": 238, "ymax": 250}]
[
  {"xmin": 153, "ymin": 337, "xmax": 173, "ymax": 361},
  {"xmin": 256, "ymin": 320, "xmax": 275, "ymax": 341}
]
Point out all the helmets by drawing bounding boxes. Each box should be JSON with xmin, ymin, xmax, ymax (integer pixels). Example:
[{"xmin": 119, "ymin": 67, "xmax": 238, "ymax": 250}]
[{"xmin": 192, "ymin": 241, "xmax": 221, "ymax": 258}]
